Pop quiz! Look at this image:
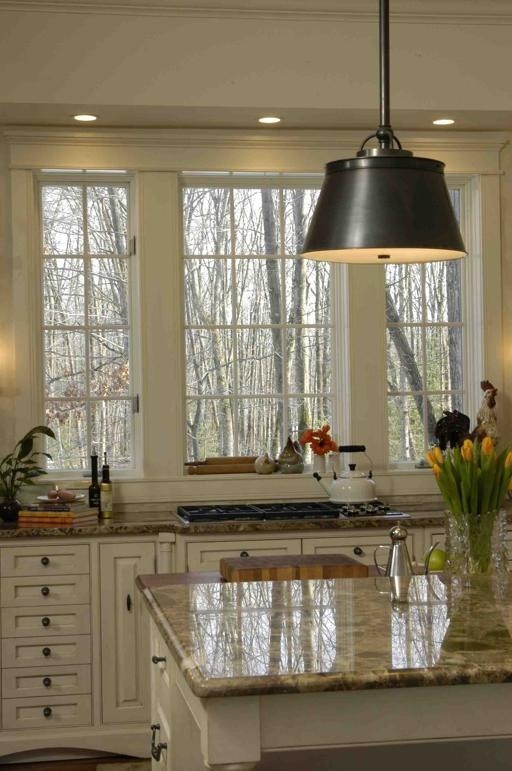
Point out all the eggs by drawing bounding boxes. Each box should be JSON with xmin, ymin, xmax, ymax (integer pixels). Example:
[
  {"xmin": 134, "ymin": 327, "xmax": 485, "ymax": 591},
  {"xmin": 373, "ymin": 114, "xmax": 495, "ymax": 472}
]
[{"xmin": 47, "ymin": 490, "xmax": 73, "ymax": 499}]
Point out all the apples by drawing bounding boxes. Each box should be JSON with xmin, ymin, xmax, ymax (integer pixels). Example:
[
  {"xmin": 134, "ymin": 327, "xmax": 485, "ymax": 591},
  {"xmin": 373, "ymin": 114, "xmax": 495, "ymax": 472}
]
[{"xmin": 424, "ymin": 547, "xmax": 447, "ymax": 571}]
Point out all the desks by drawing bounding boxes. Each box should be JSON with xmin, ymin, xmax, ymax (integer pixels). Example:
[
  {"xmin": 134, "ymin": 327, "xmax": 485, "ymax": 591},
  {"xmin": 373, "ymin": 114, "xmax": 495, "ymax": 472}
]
[{"xmin": 136, "ymin": 561, "xmax": 512, "ymax": 770}]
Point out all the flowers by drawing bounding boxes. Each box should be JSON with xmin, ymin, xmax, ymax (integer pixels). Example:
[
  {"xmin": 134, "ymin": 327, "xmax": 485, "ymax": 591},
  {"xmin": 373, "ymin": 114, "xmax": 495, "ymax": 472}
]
[
  {"xmin": 299, "ymin": 425, "xmax": 341, "ymax": 456},
  {"xmin": 424, "ymin": 436, "xmax": 512, "ymax": 570}
]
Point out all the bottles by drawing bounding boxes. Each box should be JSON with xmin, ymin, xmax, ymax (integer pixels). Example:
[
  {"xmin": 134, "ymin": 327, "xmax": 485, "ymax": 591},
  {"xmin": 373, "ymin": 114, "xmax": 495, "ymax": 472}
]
[
  {"xmin": 88, "ymin": 455, "xmax": 100, "ymax": 508},
  {"xmin": 99, "ymin": 465, "xmax": 113, "ymax": 520}
]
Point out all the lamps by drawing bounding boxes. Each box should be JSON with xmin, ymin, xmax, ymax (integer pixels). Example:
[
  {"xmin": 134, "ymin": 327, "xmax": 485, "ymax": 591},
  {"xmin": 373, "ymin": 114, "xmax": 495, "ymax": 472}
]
[{"xmin": 299, "ymin": 1, "xmax": 469, "ymax": 264}]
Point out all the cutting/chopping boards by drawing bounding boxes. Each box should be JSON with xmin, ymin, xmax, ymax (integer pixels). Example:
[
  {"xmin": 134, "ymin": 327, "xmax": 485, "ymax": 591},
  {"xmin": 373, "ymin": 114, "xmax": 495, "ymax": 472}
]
[{"xmin": 218, "ymin": 553, "xmax": 369, "ymax": 584}]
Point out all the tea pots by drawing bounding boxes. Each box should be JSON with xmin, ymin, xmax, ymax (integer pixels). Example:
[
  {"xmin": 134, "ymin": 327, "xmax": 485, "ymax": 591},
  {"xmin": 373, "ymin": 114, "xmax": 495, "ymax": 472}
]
[
  {"xmin": 373, "ymin": 519, "xmax": 441, "ymax": 576},
  {"xmin": 373, "ymin": 576, "xmax": 443, "ymax": 613},
  {"xmin": 311, "ymin": 445, "xmax": 379, "ymax": 505}
]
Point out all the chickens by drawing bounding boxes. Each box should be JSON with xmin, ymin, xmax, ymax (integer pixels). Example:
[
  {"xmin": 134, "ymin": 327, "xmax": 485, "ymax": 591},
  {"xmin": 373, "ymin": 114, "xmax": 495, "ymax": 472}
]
[{"xmin": 434, "ymin": 380, "xmax": 498, "ymax": 451}]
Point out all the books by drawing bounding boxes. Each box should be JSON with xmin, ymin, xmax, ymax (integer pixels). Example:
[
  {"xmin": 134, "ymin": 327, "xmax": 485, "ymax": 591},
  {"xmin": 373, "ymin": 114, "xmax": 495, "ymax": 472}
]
[{"xmin": 18, "ymin": 501, "xmax": 99, "ymax": 530}]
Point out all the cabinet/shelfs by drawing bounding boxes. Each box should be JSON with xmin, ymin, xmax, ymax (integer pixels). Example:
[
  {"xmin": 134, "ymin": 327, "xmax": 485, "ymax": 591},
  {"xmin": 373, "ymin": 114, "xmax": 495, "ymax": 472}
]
[
  {"xmin": 96, "ymin": 533, "xmax": 182, "ymax": 728},
  {"xmin": 0, "ymin": 528, "xmax": 99, "ymax": 730},
  {"xmin": 180, "ymin": 526, "xmax": 424, "ymax": 573}
]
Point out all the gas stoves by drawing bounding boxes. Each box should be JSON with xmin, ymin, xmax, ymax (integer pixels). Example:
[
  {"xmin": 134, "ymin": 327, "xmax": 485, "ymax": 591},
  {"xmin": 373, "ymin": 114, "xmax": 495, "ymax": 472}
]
[{"xmin": 171, "ymin": 498, "xmax": 411, "ymax": 526}]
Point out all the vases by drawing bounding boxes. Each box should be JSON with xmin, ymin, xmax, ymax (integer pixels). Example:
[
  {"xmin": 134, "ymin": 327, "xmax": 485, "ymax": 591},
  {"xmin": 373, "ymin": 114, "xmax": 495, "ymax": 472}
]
[
  {"xmin": 312, "ymin": 452, "xmax": 326, "ymax": 477},
  {"xmin": 439, "ymin": 507, "xmax": 506, "ymax": 573}
]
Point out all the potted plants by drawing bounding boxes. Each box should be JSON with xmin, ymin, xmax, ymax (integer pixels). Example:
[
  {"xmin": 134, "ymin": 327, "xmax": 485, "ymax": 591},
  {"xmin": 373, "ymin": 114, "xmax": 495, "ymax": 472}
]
[{"xmin": 0, "ymin": 425, "xmax": 60, "ymax": 522}]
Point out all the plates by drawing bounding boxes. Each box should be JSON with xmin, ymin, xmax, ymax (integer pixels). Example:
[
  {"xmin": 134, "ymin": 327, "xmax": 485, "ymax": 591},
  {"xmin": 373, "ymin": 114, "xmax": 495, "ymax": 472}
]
[{"xmin": 36, "ymin": 494, "xmax": 85, "ymax": 502}]
